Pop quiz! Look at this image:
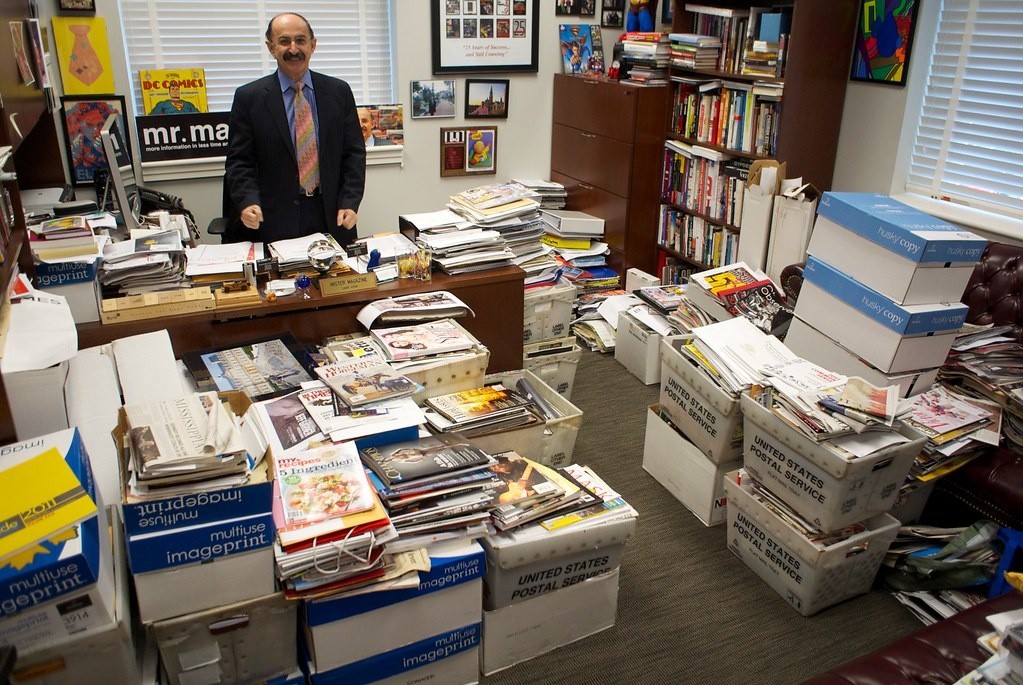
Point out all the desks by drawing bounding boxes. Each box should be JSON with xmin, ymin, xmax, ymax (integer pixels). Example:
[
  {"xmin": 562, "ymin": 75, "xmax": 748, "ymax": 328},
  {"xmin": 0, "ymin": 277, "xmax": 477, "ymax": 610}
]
[{"xmin": 75, "ymin": 260, "xmax": 529, "ymax": 373}]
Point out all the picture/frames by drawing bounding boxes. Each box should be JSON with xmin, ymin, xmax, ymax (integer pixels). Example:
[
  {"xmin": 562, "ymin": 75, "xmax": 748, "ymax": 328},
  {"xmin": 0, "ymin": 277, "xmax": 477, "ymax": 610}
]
[
  {"xmin": 661, "ymin": 0, "xmax": 672, "ymax": 23},
  {"xmin": 555, "ymin": 0, "xmax": 596, "ymax": 16},
  {"xmin": 601, "ymin": 0, "xmax": 626, "ymax": 28},
  {"xmin": 60, "ymin": 95, "xmax": 133, "ymax": 186},
  {"xmin": 431, "ymin": 0, "xmax": 540, "ymax": 76},
  {"xmin": 850, "ymin": 0, "xmax": 921, "ymax": 86},
  {"xmin": 411, "ymin": 79, "xmax": 457, "ymax": 121},
  {"xmin": 440, "ymin": 125, "xmax": 499, "ymax": 177},
  {"xmin": 464, "ymin": 79, "xmax": 512, "ymax": 118}
]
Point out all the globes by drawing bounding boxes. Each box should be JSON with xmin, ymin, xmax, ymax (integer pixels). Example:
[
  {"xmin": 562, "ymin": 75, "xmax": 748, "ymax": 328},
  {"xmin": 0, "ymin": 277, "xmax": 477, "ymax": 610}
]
[{"xmin": 297, "ymin": 275, "xmax": 311, "ymax": 299}]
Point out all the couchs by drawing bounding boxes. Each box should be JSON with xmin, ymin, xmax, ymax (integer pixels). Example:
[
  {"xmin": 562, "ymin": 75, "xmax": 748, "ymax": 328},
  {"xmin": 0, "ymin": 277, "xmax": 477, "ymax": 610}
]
[{"xmin": 783, "ymin": 227, "xmax": 1023, "ymax": 534}]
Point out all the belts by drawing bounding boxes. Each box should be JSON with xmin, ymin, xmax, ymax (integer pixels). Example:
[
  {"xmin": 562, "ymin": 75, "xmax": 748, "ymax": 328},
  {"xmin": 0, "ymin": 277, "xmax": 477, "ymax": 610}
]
[{"xmin": 300, "ymin": 186, "xmax": 321, "ymax": 197}]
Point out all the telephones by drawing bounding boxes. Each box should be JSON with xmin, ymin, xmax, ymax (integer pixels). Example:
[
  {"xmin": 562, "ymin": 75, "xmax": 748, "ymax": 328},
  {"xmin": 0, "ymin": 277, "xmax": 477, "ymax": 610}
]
[{"xmin": 137, "ymin": 186, "xmax": 177, "ymax": 213}]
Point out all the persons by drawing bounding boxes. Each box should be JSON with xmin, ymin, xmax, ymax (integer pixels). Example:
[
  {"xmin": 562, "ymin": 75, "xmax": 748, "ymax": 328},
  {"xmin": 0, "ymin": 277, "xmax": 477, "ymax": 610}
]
[
  {"xmin": 357, "ymin": 108, "xmax": 398, "ymax": 146},
  {"xmin": 626, "ymin": 0, "xmax": 654, "ymax": 32},
  {"xmin": 558, "ymin": 0, "xmax": 573, "ymax": 14},
  {"xmin": 429, "ymin": 96, "xmax": 436, "ymax": 116},
  {"xmin": 226, "ymin": 13, "xmax": 367, "ymax": 250},
  {"xmin": 471, "ymin": 101, "xmax": 489, "ymax": 115}
]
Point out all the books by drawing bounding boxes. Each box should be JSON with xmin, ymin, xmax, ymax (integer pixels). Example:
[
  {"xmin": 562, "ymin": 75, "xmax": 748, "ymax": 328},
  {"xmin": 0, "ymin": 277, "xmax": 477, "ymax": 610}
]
[
  {"xmin": 640, "ymin": 262, "xmax": 1023, "ymax": 685},
  {"xmin": 29, "ymin": 209, "xmax": 264, "ymax": 303},
  {"xmin": 622, "ymin": 31, "xmax": 670, "ymax": 87},
  {"xmin": 661, "ymin": 140, "xmax": 754, "ymax": 227},
  {"xmin": 662, "ymin": 256, "xmax": 696, "ymax": 285},
  {"xmin": 413, "ymin": 177, "xmax": 568, "ymax": 286},
  {"xmin": 568, "ymin": 268, "xmax": 632, "ymax": 355},
  {"xmin": 0, "ymin": 447, "xmax": 100, "ymax": 560},
  {"xmin": 271, "ymin": 319, "xmax": 630, "ymax": 602},
  {"xmin": 668, "ymin": 75, "xmax": 785, "ymax": 158},
  {"xmin": 657, "ymin": 205, "xmax": 739, "ymax": 270},
  {"xmin": 668, "ymin": 4, "xmax": 789, "ymax": 78}
]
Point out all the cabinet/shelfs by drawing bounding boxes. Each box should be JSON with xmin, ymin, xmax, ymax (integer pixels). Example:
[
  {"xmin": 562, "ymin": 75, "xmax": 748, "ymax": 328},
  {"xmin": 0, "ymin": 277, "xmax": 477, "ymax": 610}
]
[
  {"xmin": 0, "ymin": 0, "xmax": 68, "ymax": 309},
  {"xmin": 657, "ymin": 0, "xmax": 861, "ymax": 282},
  {"xmin": 547, "ymin": 73, "xmax": 667, "ymax": 289}
]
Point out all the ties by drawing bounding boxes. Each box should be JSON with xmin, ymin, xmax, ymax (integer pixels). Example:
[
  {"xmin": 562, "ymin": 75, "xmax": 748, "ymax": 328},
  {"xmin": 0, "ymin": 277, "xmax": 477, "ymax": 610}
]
[{"xmin": 291, "ymin": 81, "xmax": 320, "ymax": 194}]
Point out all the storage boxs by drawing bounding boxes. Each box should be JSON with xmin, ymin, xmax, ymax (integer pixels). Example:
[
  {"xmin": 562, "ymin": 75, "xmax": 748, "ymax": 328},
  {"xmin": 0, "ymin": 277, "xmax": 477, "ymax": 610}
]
[{"xmin": 0, "ymin": 190, "xmax": 964, "ymax": 685}]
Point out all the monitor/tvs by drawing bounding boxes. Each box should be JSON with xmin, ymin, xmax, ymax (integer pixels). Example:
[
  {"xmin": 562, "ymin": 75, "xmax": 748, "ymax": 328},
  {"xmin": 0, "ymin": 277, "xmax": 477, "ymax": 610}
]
[{"xmin": 100, "ymin": 114, "xmax": 141, "ymax": 233}]
